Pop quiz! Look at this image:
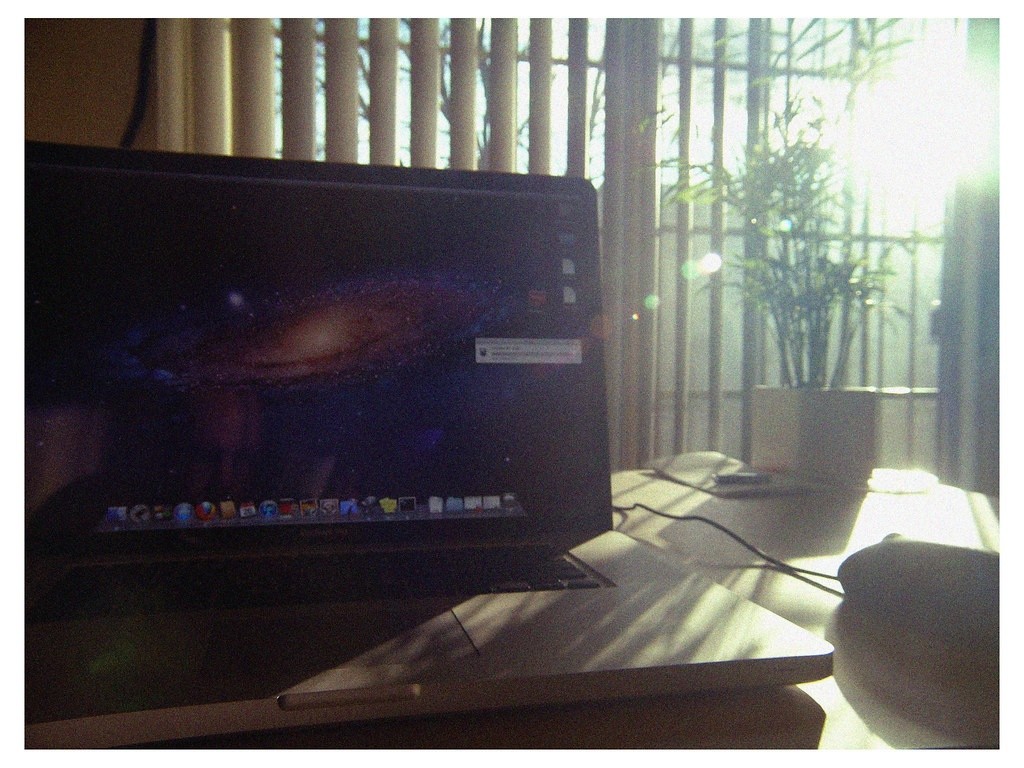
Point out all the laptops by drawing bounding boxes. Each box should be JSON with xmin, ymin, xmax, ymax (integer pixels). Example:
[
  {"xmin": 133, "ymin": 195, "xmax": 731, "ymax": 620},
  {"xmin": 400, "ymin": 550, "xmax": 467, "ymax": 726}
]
[{"xmin": 24, "ymin": 140, "xmax": 838, "ymax": 749}]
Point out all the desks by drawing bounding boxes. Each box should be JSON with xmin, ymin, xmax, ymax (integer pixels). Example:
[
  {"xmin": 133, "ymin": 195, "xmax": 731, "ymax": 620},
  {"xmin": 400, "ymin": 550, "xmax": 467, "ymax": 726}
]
[{"xmin": 123, "ymin": 463, "xmax": 998, "ymax": 750}]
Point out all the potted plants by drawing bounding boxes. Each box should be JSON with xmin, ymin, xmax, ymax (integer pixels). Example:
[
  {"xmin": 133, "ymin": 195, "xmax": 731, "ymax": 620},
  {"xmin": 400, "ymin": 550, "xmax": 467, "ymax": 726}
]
[{"xmin": 620, "ymin": 19, "xmax": 926, "ymax": 478}]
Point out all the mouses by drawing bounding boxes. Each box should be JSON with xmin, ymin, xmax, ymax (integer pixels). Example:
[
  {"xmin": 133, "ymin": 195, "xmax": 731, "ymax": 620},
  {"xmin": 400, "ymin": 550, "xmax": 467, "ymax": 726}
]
[{"xmin": 837, "ymin": 532, "xmax": 998, "ymax": 642}]
